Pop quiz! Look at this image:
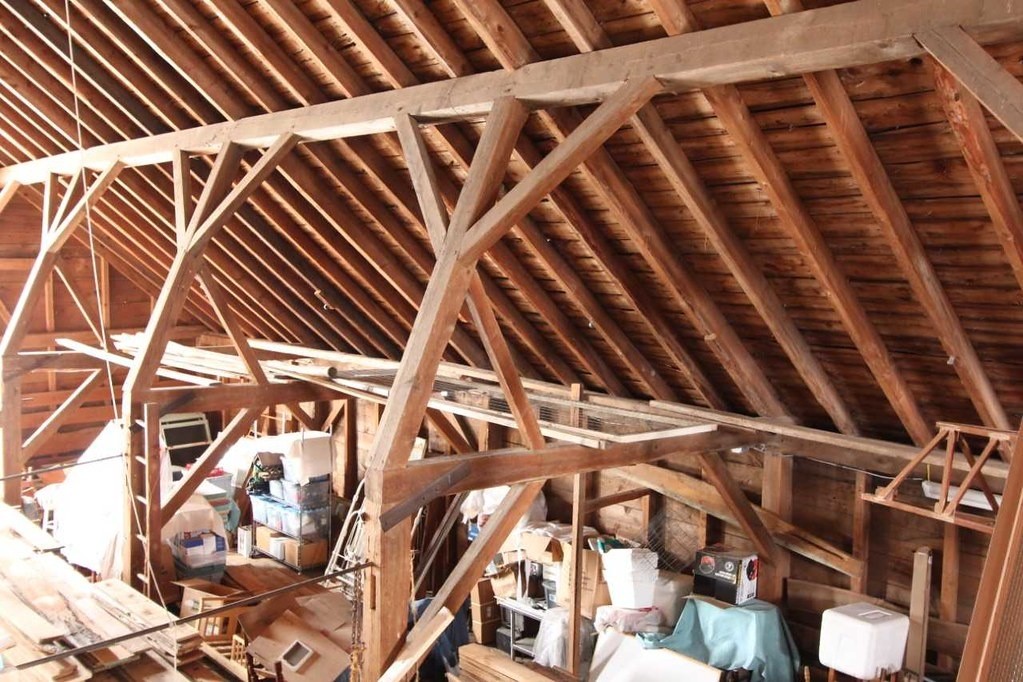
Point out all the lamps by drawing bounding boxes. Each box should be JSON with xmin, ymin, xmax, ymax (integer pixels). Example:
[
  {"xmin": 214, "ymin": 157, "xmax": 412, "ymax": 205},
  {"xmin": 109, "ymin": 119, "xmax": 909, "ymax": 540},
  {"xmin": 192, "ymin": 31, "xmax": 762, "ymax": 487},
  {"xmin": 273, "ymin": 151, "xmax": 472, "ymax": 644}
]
[{"xmin": 921, "ymin": 480, "xmax": 1003, "ymax": 511}]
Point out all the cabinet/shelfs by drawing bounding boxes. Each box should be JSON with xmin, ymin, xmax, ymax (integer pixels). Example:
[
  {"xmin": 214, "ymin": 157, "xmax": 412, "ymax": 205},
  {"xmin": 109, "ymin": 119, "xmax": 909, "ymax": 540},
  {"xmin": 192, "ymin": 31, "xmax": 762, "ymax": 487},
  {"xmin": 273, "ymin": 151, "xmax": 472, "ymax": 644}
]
[
  {"xmin": 157, "ymin": 414, "xmax": 213, "ymax": 499},
  {"xmin": 249, "ymin": 425, "xmax": 335, "ymax": 575}
]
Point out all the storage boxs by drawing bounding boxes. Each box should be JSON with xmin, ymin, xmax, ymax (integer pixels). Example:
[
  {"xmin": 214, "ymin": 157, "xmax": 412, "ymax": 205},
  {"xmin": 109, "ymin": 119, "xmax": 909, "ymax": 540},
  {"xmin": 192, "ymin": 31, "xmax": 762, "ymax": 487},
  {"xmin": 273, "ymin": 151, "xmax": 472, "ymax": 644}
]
[{"xmin": 170, "ymin": 431, "xmax": 909, "ymax": 682}]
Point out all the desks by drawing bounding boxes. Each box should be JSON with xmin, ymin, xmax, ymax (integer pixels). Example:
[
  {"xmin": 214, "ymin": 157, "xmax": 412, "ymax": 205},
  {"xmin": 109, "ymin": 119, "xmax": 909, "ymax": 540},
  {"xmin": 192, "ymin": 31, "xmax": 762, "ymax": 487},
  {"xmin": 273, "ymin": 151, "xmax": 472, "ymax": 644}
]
[
  {"xmin": 500, "ymin": 604, "xmax": 598, "ymax": 671},
  {"xmin": 636, "ymin": 593, "xmax": 800, "ymax": 682}
]
